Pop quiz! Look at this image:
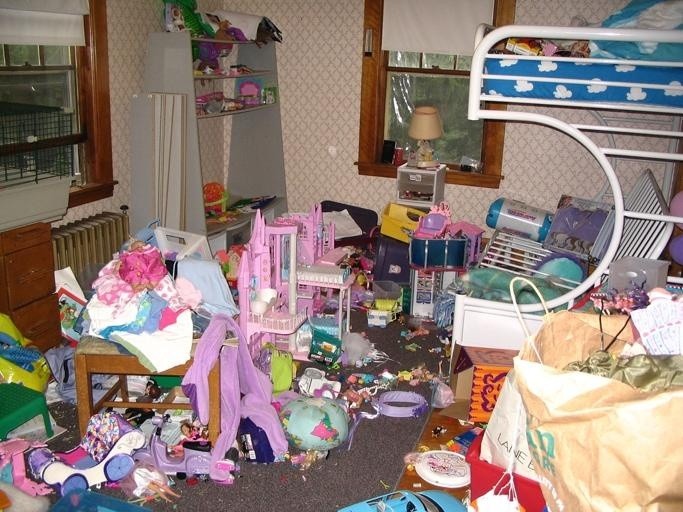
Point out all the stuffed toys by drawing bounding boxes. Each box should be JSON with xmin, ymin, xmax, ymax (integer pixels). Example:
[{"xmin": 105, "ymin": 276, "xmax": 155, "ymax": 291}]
[{"xmin": 193, "ymin": 42, "xmax": 233, "ymax": 71}]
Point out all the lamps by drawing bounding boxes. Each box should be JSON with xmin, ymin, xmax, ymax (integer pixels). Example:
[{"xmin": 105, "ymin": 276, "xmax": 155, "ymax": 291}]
[{"xmin": 407, "ymin": 105, "xmax": 443, "ymax": 168}]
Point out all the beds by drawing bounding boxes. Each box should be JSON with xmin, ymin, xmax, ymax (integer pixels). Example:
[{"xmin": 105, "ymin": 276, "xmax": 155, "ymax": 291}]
[{"xmin": 447, "ymin": 4, "xmax": 683, "ymax": 372}]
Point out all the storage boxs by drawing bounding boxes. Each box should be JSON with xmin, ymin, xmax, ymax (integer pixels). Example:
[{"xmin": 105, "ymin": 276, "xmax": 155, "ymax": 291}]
[{"xmin": 369, "ymin": 201, "xmax": 468, "ymax": 285}]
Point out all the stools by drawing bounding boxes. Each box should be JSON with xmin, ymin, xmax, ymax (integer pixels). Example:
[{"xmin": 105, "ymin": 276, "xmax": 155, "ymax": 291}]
[{"xmin": 72, "ymin": 332, "xmax": 224, "ymax": 446}]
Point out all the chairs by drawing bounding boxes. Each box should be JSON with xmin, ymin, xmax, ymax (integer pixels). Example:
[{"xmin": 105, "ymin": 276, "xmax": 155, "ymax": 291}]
[{"xmin": 0, "ymin": 316, "xmax": 55, "ymax": 441}]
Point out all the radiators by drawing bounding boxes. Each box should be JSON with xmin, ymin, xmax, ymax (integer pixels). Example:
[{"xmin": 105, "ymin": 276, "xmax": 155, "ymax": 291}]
[{"xmin": 52, "ymin": 210, "xmax": 129, "ymax": 323}]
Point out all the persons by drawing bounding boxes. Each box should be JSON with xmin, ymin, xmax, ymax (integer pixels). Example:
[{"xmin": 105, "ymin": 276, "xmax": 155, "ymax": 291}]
[{"xmin": 122, "ymin": 376, "xmax": 161, "ymax": 422}]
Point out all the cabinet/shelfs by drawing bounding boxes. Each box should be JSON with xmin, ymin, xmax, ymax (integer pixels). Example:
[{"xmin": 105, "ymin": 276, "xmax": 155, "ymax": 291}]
[
  {"xmin": 145, "ymin": 30, "xmax": 289, "ymax": 256},
  {"xmin": 1, "ymin": 223, "xmax": 66, "ymax": 351},
  {"xmin": 397, "ymin": 163, "xmax": 447, "ymax": 211}
]
